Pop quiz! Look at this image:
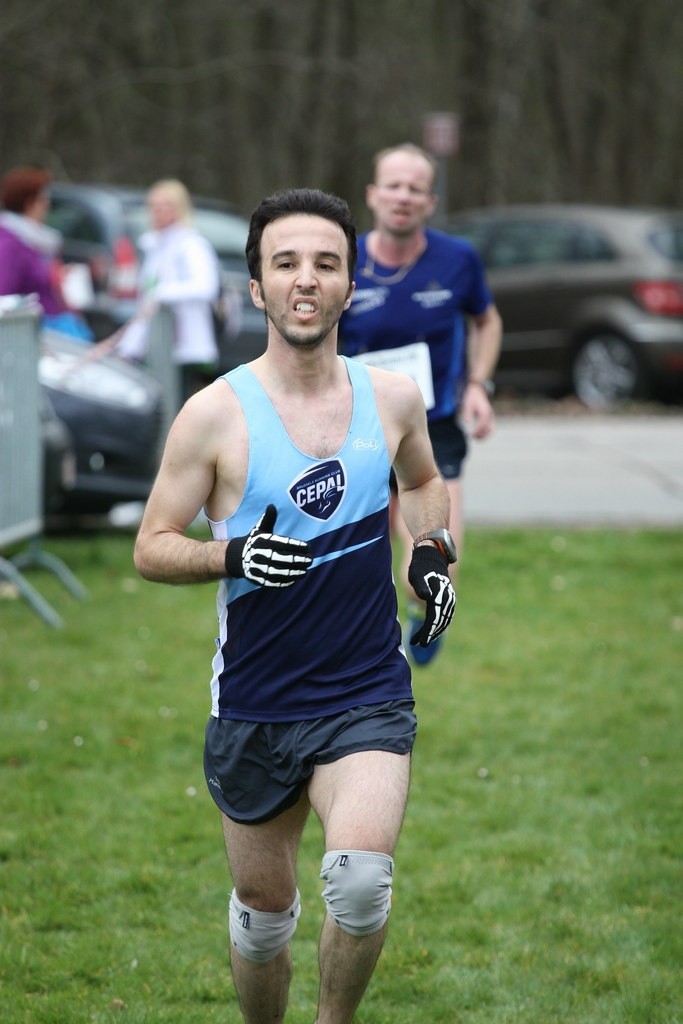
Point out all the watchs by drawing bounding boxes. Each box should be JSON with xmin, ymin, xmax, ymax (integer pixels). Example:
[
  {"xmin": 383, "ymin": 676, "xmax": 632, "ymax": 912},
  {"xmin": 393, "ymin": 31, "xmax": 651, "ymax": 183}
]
[
  {"xmin": 466, "ymin": 379, "xmax": 496, "ymax": 401},
  {"xmin": 412, "ymin": 529, "xmax": 458, "ymax": 564}
]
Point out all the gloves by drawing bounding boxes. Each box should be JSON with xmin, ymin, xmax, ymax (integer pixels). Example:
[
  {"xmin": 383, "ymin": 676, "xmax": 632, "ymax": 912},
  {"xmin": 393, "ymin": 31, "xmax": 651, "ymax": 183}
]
[
  {"xmin": 225, "ymin": 503, "xmax": 313, "ymax": 589},
  {"xmin": 408, "ymin": 545, "xmax": 456, "ymax": 648}
]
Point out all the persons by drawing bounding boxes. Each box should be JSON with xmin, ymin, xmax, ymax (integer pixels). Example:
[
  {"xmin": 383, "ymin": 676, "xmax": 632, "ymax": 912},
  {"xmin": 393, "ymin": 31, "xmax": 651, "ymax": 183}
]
[
  {"xmin": 133, "ymin": 190, "xmax": 458, "ymax": 1024},
  {"xmin": 1, "ymin": 166, "xmax": 84, "ymax": 508},
  {"xmin": 336, "ymin": 142, "xmax": 502, "ymax": 664},
  {"xmin": 73, "ymin": 182, "xmax": 221, "ymax": 523}
]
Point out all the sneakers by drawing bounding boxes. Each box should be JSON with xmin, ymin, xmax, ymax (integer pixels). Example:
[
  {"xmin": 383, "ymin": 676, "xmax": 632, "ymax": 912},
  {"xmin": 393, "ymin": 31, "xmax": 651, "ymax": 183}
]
[{"xmin": 407, "ymin": 605, "xmax": 444, "ymax": 667}]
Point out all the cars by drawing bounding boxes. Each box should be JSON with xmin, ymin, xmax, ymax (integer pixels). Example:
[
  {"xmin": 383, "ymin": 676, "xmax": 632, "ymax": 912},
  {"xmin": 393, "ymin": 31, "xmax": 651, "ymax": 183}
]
[
  {"xmin": 45, "ymin": 183, "xmax": 268, "ymax": 389},
  {"xmin": 38, "ymin": 325, "xmax": 173, "ymax": 537},
  {"xmin": 429, "ymin": 205, "xmax": 682, "ymax": 409}
]
